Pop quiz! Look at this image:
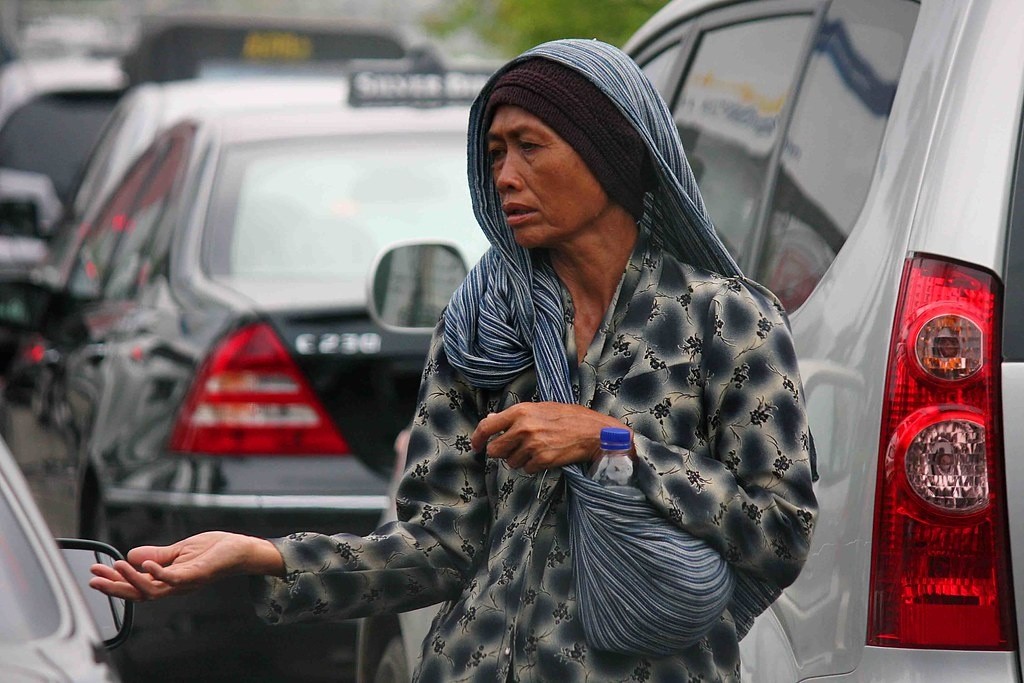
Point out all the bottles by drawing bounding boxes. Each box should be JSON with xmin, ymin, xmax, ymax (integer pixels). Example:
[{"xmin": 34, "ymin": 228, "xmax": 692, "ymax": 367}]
[{"xmin": 585, "ymin": 428, "xmax": 644, "ymax": 496}]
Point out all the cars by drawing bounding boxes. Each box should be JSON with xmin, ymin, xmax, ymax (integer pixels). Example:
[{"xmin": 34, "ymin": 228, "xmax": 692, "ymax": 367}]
[{"xmin": 0, "ymin": 1, "xmax": 1024, "ymax": 683}]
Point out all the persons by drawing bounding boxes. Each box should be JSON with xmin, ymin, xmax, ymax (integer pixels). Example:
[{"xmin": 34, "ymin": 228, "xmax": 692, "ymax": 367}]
[{"xmin": 89, "ymin": 39, "xmax": 819, "ymax": 683}]
